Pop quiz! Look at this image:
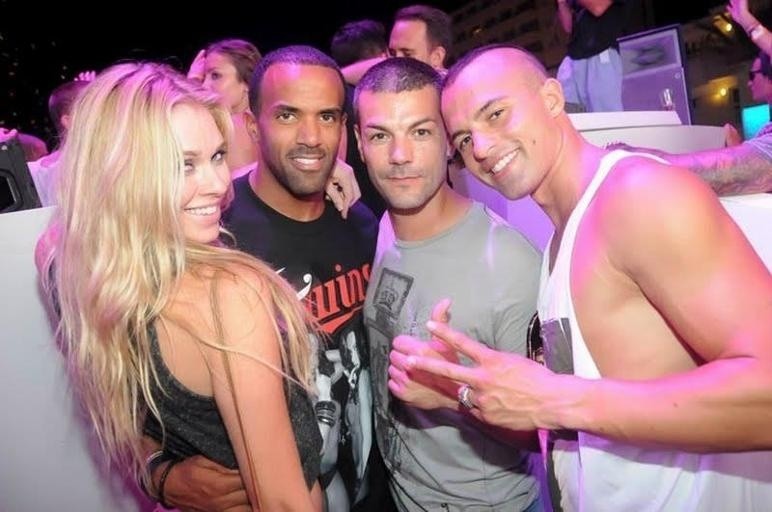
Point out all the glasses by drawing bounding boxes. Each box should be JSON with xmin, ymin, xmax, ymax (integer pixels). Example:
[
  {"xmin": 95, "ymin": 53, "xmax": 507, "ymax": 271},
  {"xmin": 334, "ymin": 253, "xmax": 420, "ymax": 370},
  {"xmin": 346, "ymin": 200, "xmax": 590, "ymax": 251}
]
[{"xmin": 749, "ymin": 69, "xmax": 760, "ymax": 80}]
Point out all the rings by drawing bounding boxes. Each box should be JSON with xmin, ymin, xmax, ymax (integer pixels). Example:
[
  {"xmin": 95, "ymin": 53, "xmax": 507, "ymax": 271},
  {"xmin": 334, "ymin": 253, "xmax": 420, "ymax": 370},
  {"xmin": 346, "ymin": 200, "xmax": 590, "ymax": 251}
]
[{"xmin": 457, "ymin": 384, "xmax": 474, "ymax": 409}]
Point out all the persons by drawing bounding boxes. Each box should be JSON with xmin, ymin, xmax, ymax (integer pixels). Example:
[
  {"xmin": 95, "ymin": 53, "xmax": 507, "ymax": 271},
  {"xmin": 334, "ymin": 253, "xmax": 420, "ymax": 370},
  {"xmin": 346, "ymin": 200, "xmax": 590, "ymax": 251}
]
[
  {"xmin": 349, "ymin": 56, "xmax": 555, "ymax": 512},
  {"xmin": 83, "ymin": 44, "xmax": 398, "ymax": 511},
  {"xmin": 387, "ymin": 42, "xmax": 771, "ymax": 512},
  {"xmin": 36, "ymin": 61, "xmax": 328, "ymax": 512},
  {"xmin": 298, "ymin": 321, "xmax": 398, "ymax": 511}
]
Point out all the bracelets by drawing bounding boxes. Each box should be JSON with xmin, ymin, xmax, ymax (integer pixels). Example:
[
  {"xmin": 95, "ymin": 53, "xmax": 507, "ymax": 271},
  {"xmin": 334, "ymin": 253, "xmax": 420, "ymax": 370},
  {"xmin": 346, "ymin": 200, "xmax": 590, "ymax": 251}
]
[{"xmin": 137, "ymin": 451, "xmax": 182, "ymax": 509}]
